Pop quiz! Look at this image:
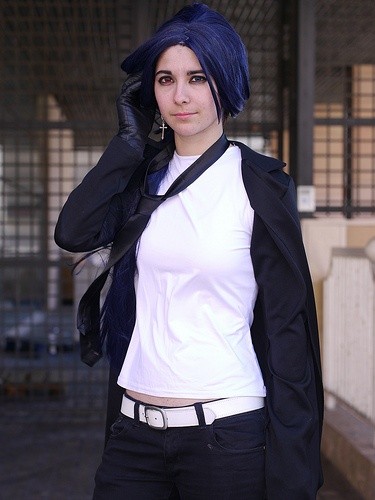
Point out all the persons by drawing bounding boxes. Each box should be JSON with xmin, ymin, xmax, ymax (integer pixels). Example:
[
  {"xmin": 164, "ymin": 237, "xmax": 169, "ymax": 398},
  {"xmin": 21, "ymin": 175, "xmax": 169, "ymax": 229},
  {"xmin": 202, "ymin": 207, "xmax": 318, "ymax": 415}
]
[{"xmin": 52, "ymin": 6, "xmax": 325, "ymax": 500}]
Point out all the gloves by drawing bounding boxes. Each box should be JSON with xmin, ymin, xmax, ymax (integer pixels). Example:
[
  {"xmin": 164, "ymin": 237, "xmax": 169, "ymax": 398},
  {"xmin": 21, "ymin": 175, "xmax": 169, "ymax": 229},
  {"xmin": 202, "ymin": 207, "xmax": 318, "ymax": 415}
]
[{"xmin": 116, "ymin": 73, "xmax": 157, "ymax": 154}]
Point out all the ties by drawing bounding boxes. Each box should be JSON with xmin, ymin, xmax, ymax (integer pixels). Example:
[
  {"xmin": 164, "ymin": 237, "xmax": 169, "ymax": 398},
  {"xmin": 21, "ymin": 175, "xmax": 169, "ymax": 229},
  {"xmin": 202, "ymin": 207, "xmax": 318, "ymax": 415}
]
[{"xmin": 78, "ymin": 134, "xmax": 229, "ymax": 365}]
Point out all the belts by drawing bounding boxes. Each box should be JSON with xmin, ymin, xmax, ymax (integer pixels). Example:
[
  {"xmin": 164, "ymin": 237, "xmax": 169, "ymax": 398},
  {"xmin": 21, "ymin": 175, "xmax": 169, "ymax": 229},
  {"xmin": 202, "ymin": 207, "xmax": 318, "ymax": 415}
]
[{"xmin": 119, "ymin": 396, "xmax": 265, "ymax": 431}]
